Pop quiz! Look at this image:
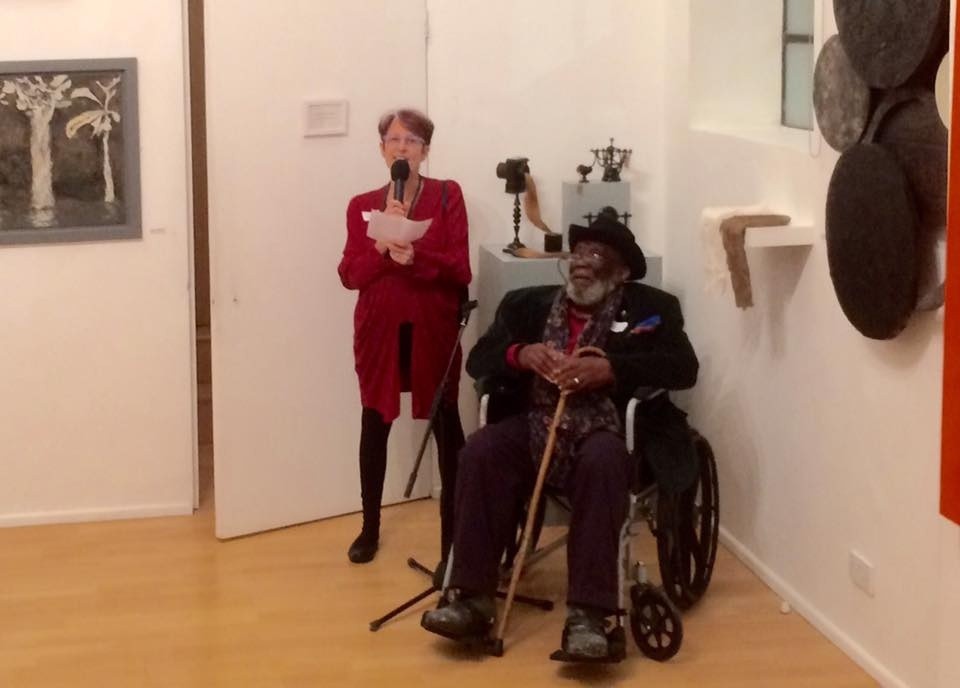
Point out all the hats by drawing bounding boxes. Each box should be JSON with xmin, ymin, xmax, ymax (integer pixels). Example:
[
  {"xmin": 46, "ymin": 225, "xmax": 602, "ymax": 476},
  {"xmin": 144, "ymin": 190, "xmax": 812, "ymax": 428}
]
[{"xmin": 568, "ymin": 217, "xmax": 647, "ymax": 281}]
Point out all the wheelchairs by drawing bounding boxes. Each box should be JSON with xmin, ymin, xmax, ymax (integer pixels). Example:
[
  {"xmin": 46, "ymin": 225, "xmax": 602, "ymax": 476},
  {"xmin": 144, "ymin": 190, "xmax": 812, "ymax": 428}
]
[{"xmin": 437, "ymin": 372, "xmax": 720, "ymax": 663}]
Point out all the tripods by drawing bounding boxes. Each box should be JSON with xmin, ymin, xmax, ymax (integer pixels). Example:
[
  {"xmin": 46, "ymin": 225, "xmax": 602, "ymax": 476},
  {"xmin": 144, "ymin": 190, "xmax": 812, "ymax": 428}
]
[{"xmin": 369, "ymin": 301, "xmax": 556, "ymax": 633}]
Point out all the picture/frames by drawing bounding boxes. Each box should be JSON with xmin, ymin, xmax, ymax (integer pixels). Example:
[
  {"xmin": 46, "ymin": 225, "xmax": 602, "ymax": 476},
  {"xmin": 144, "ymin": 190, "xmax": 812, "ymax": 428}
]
[{"xmin": 0, "ymin": 56, "xmax": 142, "ymax": 246}]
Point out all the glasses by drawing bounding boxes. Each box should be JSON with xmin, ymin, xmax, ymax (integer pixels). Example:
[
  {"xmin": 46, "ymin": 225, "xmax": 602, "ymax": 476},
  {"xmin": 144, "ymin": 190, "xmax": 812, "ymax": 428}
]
[{"xmin": 381, "ymin": 133, "xmax": 426, "ymax": 146}]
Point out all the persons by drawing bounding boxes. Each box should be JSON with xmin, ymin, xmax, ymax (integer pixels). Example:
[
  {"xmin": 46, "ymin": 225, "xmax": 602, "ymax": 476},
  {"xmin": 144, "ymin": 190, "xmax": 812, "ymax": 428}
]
[
  {"xmin": 338, "ymin": 108, "xmax": 473, "ymax": 591},
  {"xmin": 419, "ymin": 205, "xmax": 702, "ymax": 664}
]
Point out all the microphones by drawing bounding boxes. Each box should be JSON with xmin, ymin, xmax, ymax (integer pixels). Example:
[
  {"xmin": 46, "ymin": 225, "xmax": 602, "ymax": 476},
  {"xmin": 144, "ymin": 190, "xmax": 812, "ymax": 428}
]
[{"xmin": 390, "ymin": 159, "xmax": 410, "ymax": 203}]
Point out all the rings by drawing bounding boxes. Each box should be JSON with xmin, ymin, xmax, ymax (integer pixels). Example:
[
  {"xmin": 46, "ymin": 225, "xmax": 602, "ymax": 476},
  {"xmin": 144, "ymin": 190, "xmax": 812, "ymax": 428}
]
[{"xmin": 573, "ymin": 376, "xmax": 581, "ymax": 384}]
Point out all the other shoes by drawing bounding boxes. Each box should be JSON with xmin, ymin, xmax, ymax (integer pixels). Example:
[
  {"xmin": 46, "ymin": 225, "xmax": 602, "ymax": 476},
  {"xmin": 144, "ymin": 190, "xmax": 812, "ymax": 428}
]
[
  {"xmin": 349, "ymin": 535, "xmax": 377, "ymax": 564},
  {"xmin": 433, "ymin": 561, "xmax": 445, "ymax": 587},
  {"xmin": 561, "ymin": 609, "xmax": 611, "ymax": 658},
  {"xmin": 421, "ymin": 594, "xmax": 498, "ymax": 642}
]
